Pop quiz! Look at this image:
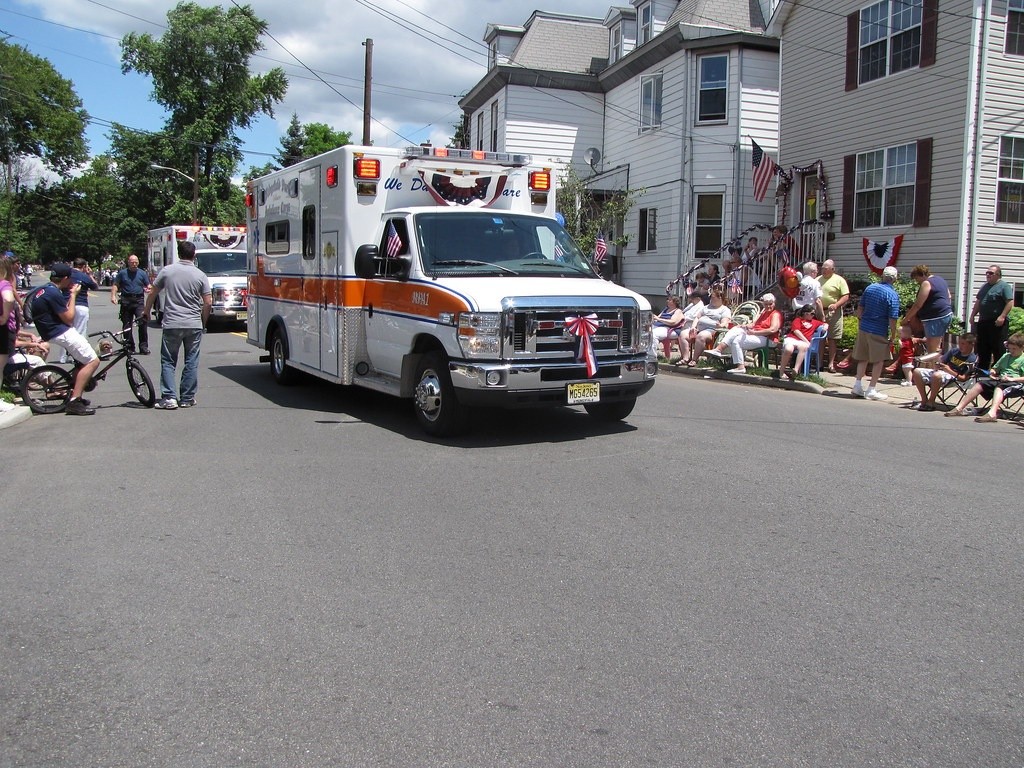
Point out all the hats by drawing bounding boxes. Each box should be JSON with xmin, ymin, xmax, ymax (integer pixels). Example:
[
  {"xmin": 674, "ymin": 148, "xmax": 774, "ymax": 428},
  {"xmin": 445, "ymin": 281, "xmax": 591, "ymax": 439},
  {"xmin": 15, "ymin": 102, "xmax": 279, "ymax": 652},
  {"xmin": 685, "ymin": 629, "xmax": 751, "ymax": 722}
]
[
  {"xmin": 51, "ymin": 264, "xmax": 72, "ymax": 279},
  {"xmin": 883, "ymin": 265, "xmax": 897, "ymax": 279},
  {"xmin": 689, "ymin": 292, "xmax": 701, "ymax": 298}
]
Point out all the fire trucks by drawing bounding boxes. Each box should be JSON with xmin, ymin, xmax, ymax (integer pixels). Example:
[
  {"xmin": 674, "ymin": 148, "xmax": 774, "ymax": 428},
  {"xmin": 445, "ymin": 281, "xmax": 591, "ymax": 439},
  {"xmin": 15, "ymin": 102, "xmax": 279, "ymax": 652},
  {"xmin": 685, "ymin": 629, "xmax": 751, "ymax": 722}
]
[
  {"xmin": 146, "ymin": 225, "xmax": 247, "ymax": 333},
  {"xmin": 245, "ymin": 143, "xmax": 661, "ymax": 439}
]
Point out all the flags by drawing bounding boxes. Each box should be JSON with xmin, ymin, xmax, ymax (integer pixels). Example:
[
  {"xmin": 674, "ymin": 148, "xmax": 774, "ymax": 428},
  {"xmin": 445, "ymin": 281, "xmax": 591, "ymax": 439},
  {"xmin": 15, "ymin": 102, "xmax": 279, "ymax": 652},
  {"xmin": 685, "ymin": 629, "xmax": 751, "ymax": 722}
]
[
  {"xmin": 387, "ymin": 222, "xmax": 402, "ymax": 257},
  {"xmin": 596, "ymin": 230, "xmax": 607, "ymax": 262},
  {"xmin": 555, "ymin": 245, "xmax": 563, "ymax": 262},
  {"xmin": 751, "ymin": 139, "xmax": 775, "ymax": 202}
]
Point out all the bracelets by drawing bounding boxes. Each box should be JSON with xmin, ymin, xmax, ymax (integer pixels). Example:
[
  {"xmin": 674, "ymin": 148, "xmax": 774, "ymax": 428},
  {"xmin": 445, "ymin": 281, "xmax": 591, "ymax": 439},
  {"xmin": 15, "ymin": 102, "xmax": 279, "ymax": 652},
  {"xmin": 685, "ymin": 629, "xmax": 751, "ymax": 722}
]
[{"xmin": 904, "ymin": 317, "xmax": 910, "ymax": 321}]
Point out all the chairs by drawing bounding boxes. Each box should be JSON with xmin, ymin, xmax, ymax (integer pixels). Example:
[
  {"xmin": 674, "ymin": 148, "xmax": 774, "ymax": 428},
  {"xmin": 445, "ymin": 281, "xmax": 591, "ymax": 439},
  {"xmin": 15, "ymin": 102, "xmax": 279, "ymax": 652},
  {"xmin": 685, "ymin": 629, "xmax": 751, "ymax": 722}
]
[
  {"xmin": 691, "ymin": 323, "xmax": 733, "ymax": 361},
  {"xmin": 794, "ymin": 326, "xmax": 828, "ymax": 380},
  {"xmin": 976, "ymin": 359, "xmax": 1024, "ymax": 421},
  {"xmin": 747, "ymin": 335, "xmax": 778, "ymax": 371},
  {"xmin": 910, "ymin": 352, "xmax": 981, "ymax": 412},
  {"xmin": 660, "ymin": 318, "xmax": 686, "ymax": 360}
]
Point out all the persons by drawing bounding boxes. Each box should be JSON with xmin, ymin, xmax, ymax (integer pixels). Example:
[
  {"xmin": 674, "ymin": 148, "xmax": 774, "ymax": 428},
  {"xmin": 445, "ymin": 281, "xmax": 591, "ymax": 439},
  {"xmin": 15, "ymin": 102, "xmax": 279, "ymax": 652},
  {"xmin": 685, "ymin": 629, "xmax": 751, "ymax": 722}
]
[
  {"xmin": 791, "ymin": 260, "xmax": 826, "ymax": 373},
  {"xmin": 111, "ymin": 254, "xmax": 153, "ymax": 355},
  {"xmin": 885, "ymin": 302, "xmax": 927, "ymax": 387},
  {"xmin": 814, "ymin": 259, "xmax": 850, "ymax": 373},
  {"xmin": 676, "ymin": 291, "xmax": 731, "ymax": 367},
  {"xmin": 0, "ymin": 252, "xmax": 50, "ymax": 397},
  {"xmin": 837, "ymin": 265, "xmax": 899, "ymax": 399},
  {"xmin": 900, "ymin": 264, "xmax": 954, "ymax": 354},
  {"xmin": 779, "ymin": 304, "xmax": 829, "ymax": 379},
  {"xmin": 943, "ymin": 329, "xmax": 1024, "ymax": 422},
  {"xmin": 502, "ymin": 234, "xmax": 521, "ymax": 258},
  {"xmin": 94, "ymin": 267, "xmax": 119, "ymax": 285},
  {"xmin": 652, "ymin": 296, "xmax": 685, "ymax": 356},
  {"xmin": 61, "ymin": 258, "xmax": 100, "ymax": 363},
  {"xmin": 912, "ymin": 333, "xmax": 978, "ymax": 412},
  {"xmin": 30, "ymin": 262, "xmax": 99, "ymax": 415},
  {"xmin": 969, "ymin": 265, "xmax": 1014, "ymax": 377},
  {"xmin": 658, "ymin": 224, "xmax": 798, "ymax": 312},
  {"xmin": 702, "ymin": 292, "xmax": 783, "ymax": 373},
  {"xmin": 141, "ymin": 241, "xmax": 213, "ymax": 409}
]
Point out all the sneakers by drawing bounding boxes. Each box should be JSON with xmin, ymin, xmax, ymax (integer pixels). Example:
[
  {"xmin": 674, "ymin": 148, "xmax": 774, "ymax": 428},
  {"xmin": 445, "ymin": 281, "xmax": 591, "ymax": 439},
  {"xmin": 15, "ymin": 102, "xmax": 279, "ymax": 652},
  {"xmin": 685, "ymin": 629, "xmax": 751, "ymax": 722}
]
[
  {"xmin": 154, "ymin": 397, "xmax": 178, "ymax": 409},
  {"xmin": 865, "ymin": 391, "xmax": 888, "ymax": 401},
  {"xmin": 181, "ymin": 396, "xmax": 197, "ymax": 407},
  {"xmin": 851, "ymin": 386, "xmax": 866, "ymax": 396}
]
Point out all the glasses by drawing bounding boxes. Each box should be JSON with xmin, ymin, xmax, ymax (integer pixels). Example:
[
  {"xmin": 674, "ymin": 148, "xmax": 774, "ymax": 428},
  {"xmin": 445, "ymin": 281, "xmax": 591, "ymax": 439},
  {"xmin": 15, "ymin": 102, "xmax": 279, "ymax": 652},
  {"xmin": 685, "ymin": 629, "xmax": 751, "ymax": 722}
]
[{"xmin": 986, "ymin": 271, "xmax": 1000, "ymax": 276}]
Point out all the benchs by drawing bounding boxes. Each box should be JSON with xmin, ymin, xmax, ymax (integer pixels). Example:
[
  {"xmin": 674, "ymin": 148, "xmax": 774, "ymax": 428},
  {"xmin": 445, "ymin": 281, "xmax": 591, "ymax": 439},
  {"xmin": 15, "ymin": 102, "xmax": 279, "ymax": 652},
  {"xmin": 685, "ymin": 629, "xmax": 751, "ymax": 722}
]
[{"xmin": 4, "ymin": 345, "xmax": 33, "ymax": 391}]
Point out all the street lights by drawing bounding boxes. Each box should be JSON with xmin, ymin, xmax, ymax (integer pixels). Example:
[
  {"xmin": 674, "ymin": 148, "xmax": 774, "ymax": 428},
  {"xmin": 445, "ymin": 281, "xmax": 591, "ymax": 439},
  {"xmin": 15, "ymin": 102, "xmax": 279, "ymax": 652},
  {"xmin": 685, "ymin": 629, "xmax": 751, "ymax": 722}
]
[{"xmin": 149, "ymin": 153, "xmax": 199, "ymax": 225}]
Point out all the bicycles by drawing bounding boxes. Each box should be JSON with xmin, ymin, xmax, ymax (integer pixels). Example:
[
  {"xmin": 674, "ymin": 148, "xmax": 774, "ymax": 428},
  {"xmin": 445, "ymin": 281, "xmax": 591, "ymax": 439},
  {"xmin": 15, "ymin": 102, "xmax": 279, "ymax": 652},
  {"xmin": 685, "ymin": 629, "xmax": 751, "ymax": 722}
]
[{"xmin": 20, "ymin": 316, "xmax": 157, "ymax": 415}]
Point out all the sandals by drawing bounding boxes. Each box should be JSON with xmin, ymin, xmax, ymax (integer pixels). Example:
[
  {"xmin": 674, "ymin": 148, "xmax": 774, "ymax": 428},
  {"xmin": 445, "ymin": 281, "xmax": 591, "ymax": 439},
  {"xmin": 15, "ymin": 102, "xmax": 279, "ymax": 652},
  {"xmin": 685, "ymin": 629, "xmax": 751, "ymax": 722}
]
[
  {"xmin": 688, "ymin": 359, "xmax": 698, "ymax": 367},
  {"xmin": 675, "ymin": 358, "xmax": 690, "ymax": 365}
]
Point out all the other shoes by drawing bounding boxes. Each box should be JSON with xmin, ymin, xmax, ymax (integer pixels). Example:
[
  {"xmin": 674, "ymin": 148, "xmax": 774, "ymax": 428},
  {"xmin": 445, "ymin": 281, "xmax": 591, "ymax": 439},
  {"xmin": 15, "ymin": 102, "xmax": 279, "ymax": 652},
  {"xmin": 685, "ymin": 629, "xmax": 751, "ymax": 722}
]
[
  {"xmin": 901, "ymin": 380, "xmax": 912, "ymax": 387},
  {"xmin": 828, "ymin": 365, "xmax": 836, "ymax": 372},
  {"xmin": 704, "ymin": 348, "xmax": 722, "ymax": 358},
  {"xmin": 47, "ymin": 390, "xmax": 73, "ymax": 399},
  {"xmin": 82, "ymin": 398, "xmax": 91, "ymax": 405},
  {"xmin": 130, "ymin": 349, "xmax": 136, "ymax": 354},
  {"xmin": 140, "ymin": 349, "xmax": 151, "ymax": 354},
  {"xmin": 728, "ymin": 367, "xmax": 746, "ymax": 373},
  {"xmin": 909, "ymin": 402, "xmax": 935, "ymax": 411},
  {"xmin": 974, "ymin": 413, "xmax": 996, "ymax": 423},
  {"xmin": 66, "ymin": 399, "xmax": 95, "ymax": 415},
  {"xmin": 885, "ymin": 365, "xmax": 900, "ymax": 372},
  {"xmin": 779, "ymin": 373, "xmax": 789, "ymax": 379},
  {"xmin": 837, "ymin": 362, "xmax": 851, "ymax": 368},
  {"xmin": 945, "ymin": 409, "xmax": 963, "ymax": 416}
]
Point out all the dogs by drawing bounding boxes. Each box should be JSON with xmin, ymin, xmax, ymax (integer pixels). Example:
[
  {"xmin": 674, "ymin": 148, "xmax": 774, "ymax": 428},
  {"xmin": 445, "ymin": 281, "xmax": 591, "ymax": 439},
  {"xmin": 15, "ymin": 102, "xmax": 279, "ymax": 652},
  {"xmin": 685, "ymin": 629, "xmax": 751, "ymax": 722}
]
[{"xmin": 95, "ymin": 339, "xmax": 113, "ymax": 360}]
